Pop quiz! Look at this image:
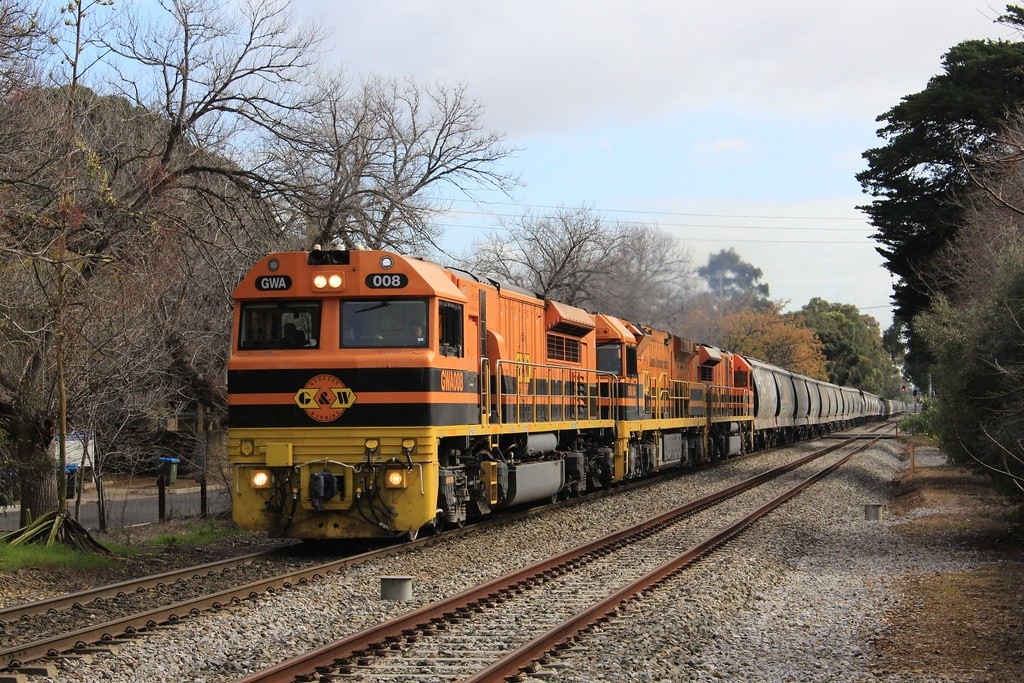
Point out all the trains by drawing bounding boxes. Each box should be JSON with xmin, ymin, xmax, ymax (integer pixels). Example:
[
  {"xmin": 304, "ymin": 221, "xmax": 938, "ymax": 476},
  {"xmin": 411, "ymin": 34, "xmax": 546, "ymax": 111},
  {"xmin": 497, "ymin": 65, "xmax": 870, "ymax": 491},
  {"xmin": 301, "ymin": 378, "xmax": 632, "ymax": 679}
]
[{"xmin": 227, "ymin": 246, "xmax": 904, "ymax": 543}]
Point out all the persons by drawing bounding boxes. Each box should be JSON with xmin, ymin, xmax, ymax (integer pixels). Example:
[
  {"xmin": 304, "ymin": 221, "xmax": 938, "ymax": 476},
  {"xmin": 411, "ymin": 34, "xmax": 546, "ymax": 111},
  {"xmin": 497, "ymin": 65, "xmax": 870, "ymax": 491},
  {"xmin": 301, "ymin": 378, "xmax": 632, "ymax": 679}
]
[
  {"xmin": 401, "ymin": 319, "xmax": 427, "ymax": 346},
  {"xmin": 268, "ymin": 322, "xmax": 311, "ymax": 349}
]
